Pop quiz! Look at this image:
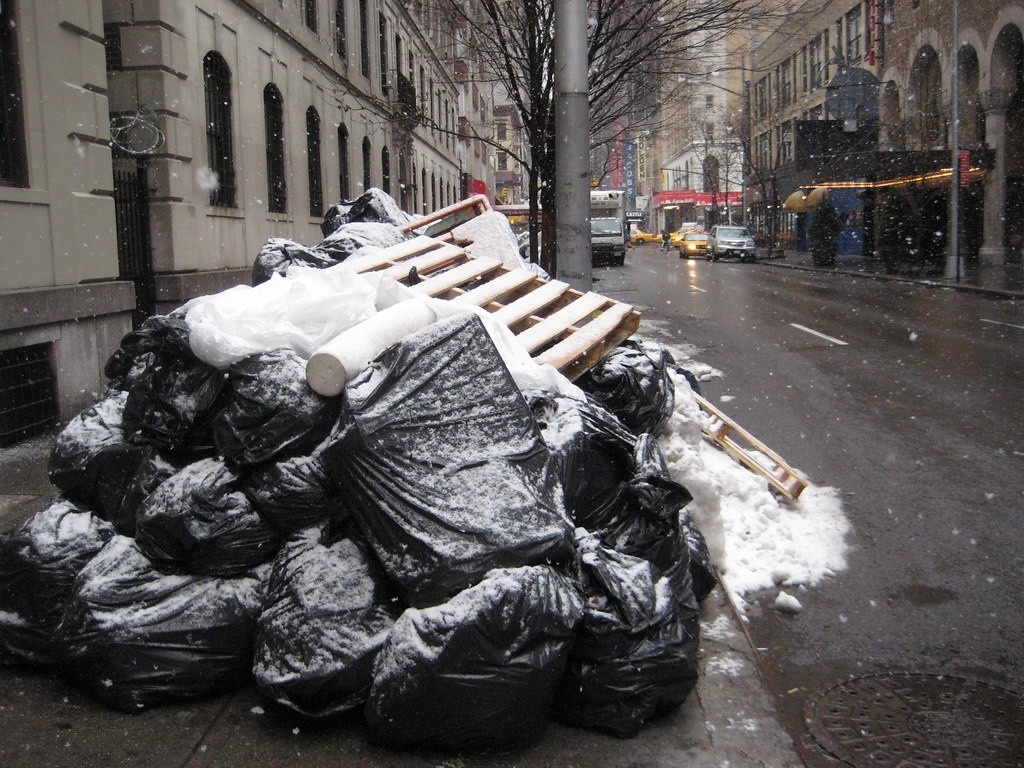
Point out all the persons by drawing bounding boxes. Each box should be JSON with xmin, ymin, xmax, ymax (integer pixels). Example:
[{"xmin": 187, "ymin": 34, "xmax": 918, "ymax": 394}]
[
  {"xmin": 747, "ymin": 219, "xmax": 757, "ymax": 246},
  {"xmin": 660, "ymin": 227, "xmax": 671, "ymax": 252},
  {"xmin": 626, "ymin": 229, "xmax": 635, "ymax": 252}
]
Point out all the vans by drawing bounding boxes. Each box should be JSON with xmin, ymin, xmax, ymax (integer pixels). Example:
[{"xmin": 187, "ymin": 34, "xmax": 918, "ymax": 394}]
[{"xmin": 589, "ymin": 214, "xmax": 627, "ymax": 265}]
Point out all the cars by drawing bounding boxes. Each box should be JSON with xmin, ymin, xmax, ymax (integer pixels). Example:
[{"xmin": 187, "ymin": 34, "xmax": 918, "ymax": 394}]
[
  {"xmin": 680, "ymin": 230, "xmax": 712, "ymax": 259},
  {"xmin": 705, "ymin": 224, "xmax": 757, "ymax": 262},
  {"xmin": 670, "ymin": 222, "xmax": 705, "ymax": 249},
  {"xmin": 625, "ymin": 227, "xmax": 656, "ymax": 246}
]
